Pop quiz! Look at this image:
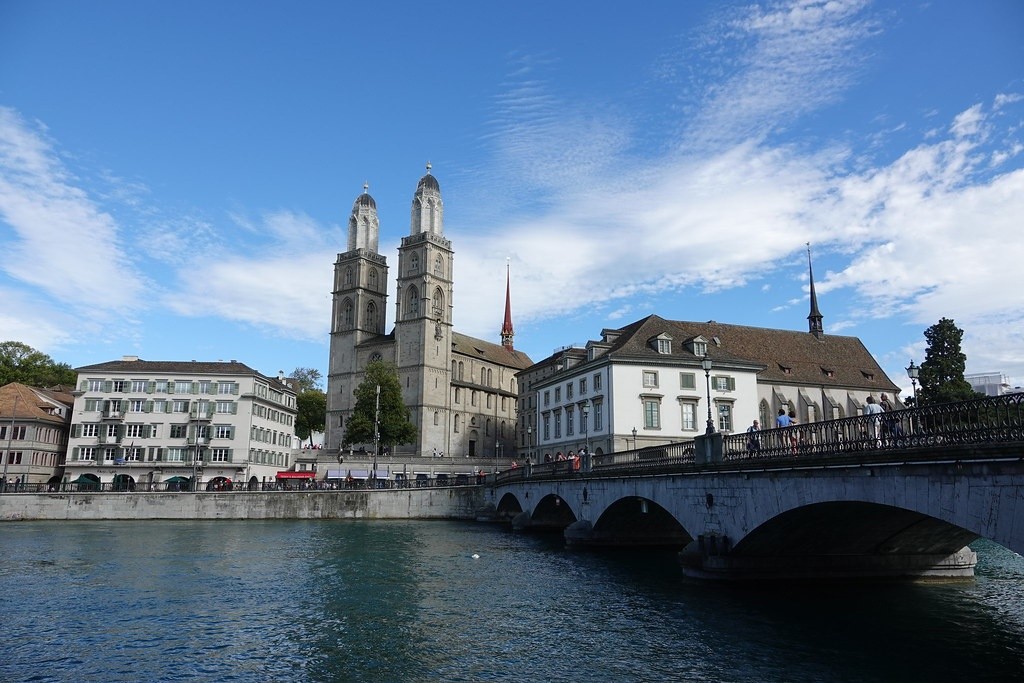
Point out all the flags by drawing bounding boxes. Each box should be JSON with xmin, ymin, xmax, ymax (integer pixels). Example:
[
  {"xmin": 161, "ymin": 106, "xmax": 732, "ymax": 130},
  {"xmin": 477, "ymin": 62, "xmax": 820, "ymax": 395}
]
[{"xmin": 125, "ymin": 442, "xmax": 132, "ymax": 461}]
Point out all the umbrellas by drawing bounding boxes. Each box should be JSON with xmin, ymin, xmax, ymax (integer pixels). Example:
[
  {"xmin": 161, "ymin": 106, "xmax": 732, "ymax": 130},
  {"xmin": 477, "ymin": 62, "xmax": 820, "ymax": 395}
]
[
  {"xmin": 164, "ymin": 476, "xmax": 187, "ymax": 483},
  {"xmin": 119, "ymin": 474, "xmax": 122, "ymax": 483},
  {"xmin": 113, "ymin": 474, "xmax": 117, "ymax": 486},
  {"xmin": 70, "ymin": 474, "xmax": 97, "ymax": 484}
]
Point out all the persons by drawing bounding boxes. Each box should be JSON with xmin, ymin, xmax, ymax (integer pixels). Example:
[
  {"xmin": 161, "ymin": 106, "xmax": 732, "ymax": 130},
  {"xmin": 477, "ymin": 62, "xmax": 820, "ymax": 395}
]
[
  {"xmin": 267, "ymin": 478, "xmax": 287, "ymax": 491},
  {"xmin": 208, "ymin": 479, "xmax": 242, "ymax": 491},
  {"xmin": 479, "ymin": 469, "xmax": 485, "ymax": 476},
  {"xmin": 776, "ymin": 409, "xmax": 800, "ymax": 454},
  {"xmin": 864, "ymin": 392, "xmax": 898, "ymax": 448},
  {"xmin": 175, "ymin": 481, "xmax": 180, "ymax": 491},
  {"xmin": 512, "ymin": 461, "xmax": 518, "ymax": 468},
  {"xmin": 747, "ymin": 420, "xmax": 760, "ymax": 458},
  {"xmin": 544, "ymin": 448, "xmax": 585, "ymax": 461},
  {"xmin": 304, "ymin": 444, "xmax": 323, "ymax": 450},
  {"xmin": 151, "ymin": 481, "xmax": 156, "ymax": 492},
  {"xmin": 433, "ymin": 448, "xmax": 443, "ymax": 457},
  {"xmin": 345, "ymin": 477, "xmax": 353, "ymax": 489},
  {"xmin": 9, "ymin": 476, "xmax": 20, "ymax": 493}
]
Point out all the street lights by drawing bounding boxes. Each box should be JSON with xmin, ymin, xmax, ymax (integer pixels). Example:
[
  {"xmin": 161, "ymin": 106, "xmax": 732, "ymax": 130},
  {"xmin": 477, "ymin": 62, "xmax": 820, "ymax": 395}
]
[
  {"xmin": 581, "ymin": 400, "xmax": 590, "ymax": 454},
  {"xmin": 526, "ymin": 424, "xmax": 533, "ymax": 464},
  {"xmin": 905, "ymin": 358, "xmax": 923, "ymax": 430},
  {"xmin": 700, "ymin": 352, "xmax": 716, "ymax": 433},
  {"xmin": 495, "ymin": 439, "xmax": 501, "ymax": 473},
  {"xmin": 632, "ymin": 427, "xmax": 638, "ymax": 461}
]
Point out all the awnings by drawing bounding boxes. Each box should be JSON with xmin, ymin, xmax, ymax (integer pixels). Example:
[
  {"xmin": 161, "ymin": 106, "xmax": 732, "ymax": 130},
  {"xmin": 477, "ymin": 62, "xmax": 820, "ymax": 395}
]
[
  {"xmin": 277, "ymin": 471, "xmax": 315, "ymax": 479},
  {"xmin": 327, "ymin": 468, "xmax": 390, "ymax": 479}
]
